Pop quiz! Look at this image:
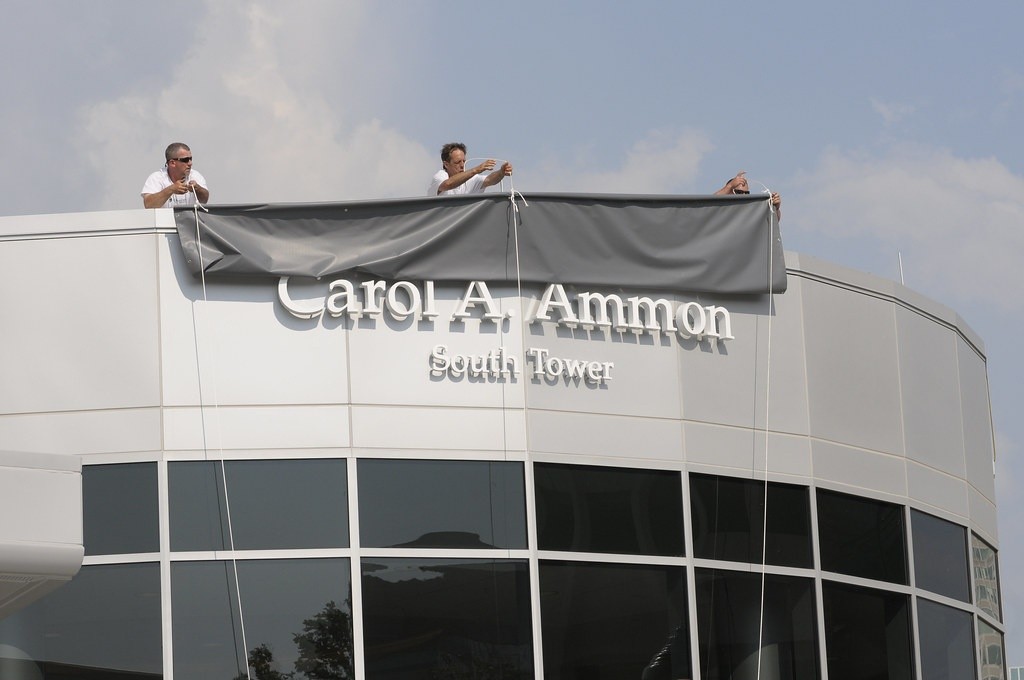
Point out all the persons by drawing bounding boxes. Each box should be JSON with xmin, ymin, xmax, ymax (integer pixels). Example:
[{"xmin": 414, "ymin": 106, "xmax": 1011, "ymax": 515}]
[
  {"xmin": 427, "ymin": 143, "xmax": 512, "ymax": 196},
  {"xmin": 141, "ymin": 143, "xmax": 209, "ymax": 208},
  {"xmin": 714, "ymin": 171, "xmax": 781, "ymax": 223}
]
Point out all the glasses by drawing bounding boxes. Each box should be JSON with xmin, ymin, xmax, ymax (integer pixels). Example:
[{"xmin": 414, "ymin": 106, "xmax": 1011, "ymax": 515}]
[
  {"xmin": 732, "ymin": 188, "xmax": 750, "ymax": 196},
  {"xmin": 167, "ymin": 156, "xmax": 193, "ymax": 163}
]
